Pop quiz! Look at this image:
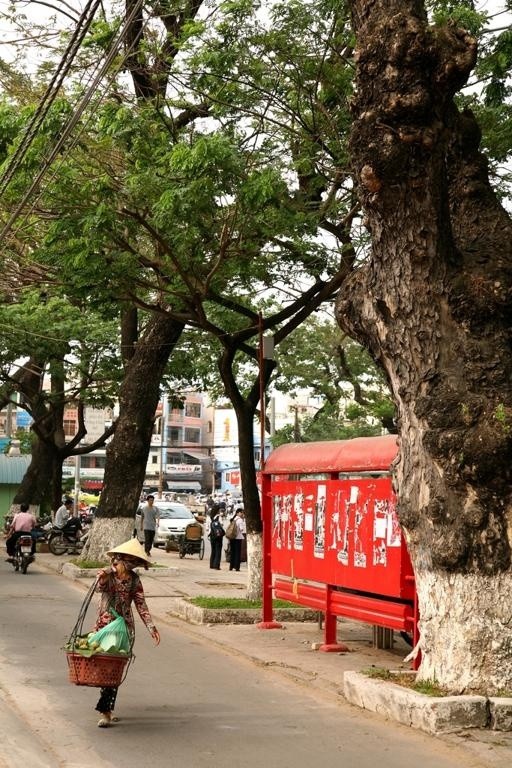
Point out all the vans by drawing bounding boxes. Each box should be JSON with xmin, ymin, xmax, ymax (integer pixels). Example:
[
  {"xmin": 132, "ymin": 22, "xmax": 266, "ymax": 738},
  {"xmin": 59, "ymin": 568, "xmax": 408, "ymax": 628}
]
[{"xmin": 134, "ymin": 502, "xmax": 200, "ymax": 547}]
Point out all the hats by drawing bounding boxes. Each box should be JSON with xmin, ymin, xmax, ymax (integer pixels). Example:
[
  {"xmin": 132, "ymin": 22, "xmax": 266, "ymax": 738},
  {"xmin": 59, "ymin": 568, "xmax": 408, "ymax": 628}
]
[{"xmin": 107, "ymin": 538, "xmax": 155, "ymax": 567}]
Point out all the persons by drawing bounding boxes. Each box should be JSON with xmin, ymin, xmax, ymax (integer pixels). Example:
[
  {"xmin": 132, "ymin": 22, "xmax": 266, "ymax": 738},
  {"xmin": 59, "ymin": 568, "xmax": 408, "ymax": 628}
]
[
  {"xmin": 54, "ymin": 498, "xmax": 89, "ymax": 534},
  {"xmin": 94, "ymin": 553, "xmax": 160, "ymax": 728},
  {"xmin": 79, "ymin": 499, "xmax": 88, "ymax": 513},
  {"xmin": 5, "ymin": 504, "xmax": 38, "ymax": 563},
  {"xmin": 61, "ymin": 490, "xmax": 74, "ymax": 516},
  {"xmin": 140, "ymin": 496, "xmax": 161, "ymax": 557},
  {"xmin": 202, "ymin": 491, "xmax": 248, "ymax": 573}
]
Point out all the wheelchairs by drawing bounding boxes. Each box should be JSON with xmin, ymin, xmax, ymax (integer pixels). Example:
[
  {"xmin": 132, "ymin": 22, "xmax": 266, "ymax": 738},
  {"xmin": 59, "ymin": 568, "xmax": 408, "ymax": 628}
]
[{"xmin": 179, "ymin": 522, "xmax": 204, "ymax": 560}]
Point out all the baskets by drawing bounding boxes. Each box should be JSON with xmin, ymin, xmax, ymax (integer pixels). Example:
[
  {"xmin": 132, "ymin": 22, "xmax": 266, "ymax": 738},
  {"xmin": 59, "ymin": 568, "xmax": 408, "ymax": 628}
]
[{"xmin": 65, "ymin": 653, "xmax": 128, "ymax": 687}]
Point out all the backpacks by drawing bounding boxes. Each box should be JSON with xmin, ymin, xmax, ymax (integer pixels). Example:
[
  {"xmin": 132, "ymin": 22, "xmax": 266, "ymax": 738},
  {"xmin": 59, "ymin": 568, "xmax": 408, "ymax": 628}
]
[
  {"xmin": 211, "ymin": 515, "xmax": 225, "ymax": 539},
  {"xmin": 226, "ymin": 518, "xmax": 241, "ymax": 539}
]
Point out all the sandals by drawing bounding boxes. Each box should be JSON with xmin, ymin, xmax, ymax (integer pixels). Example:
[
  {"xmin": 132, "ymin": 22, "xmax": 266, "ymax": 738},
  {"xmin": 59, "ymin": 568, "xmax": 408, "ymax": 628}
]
[{"xmin": 98, "ymin": 710, "xmax": 120, "ymax": 727}]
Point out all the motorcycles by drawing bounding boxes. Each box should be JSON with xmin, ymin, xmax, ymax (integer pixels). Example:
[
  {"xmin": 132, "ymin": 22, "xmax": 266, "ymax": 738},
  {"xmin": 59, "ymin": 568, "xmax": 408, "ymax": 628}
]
[
  {"xmin": 48, "ymin": 524, "xmax": 90, "ymax": 555},
  {"xmin": 5, "ymin": 522, "xmax": 35, "ymax": 574}
]
